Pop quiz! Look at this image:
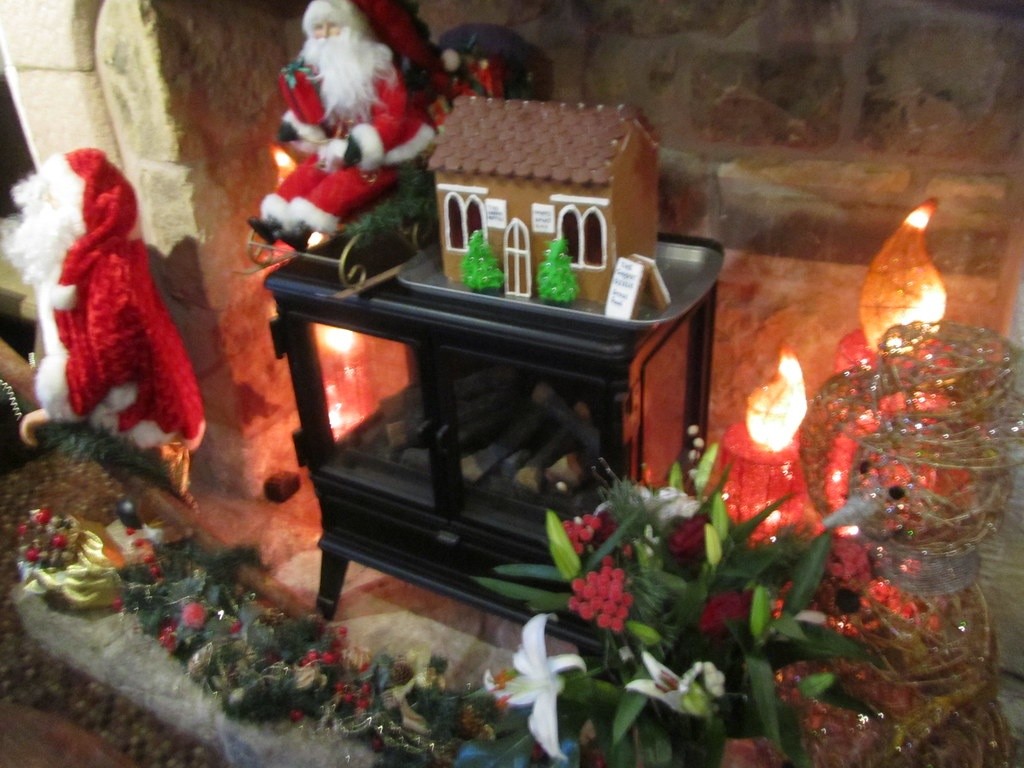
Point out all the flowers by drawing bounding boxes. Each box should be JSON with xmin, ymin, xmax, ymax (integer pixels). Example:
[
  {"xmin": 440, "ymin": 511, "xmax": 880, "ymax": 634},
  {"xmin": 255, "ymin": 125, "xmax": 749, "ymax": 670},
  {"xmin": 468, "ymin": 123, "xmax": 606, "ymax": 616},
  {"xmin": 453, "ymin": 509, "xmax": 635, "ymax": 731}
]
[{"xmin": 484, "ymin": 426, "xmax": 886, "ymax": 768}]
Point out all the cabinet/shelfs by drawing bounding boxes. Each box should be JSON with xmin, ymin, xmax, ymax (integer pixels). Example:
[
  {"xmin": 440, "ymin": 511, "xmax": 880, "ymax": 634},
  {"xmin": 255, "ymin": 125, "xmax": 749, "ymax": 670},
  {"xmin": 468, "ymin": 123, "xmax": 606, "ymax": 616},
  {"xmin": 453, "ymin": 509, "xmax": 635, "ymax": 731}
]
[{"xmin": 263, "ymin": 223, "xmax": 720, "ymax": 668}]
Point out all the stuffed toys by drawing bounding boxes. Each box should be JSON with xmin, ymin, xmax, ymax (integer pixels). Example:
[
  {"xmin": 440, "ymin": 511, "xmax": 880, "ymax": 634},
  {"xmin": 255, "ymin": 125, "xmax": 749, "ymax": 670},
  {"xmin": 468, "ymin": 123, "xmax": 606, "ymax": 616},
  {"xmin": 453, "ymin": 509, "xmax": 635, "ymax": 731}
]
[
  {"xmin": 248, "ymin": 1, "xmax": 432, "ymax": 249},
  {"xmin": 8, "ymin": 154, "xmax": 208, "ymax": 485}
]
